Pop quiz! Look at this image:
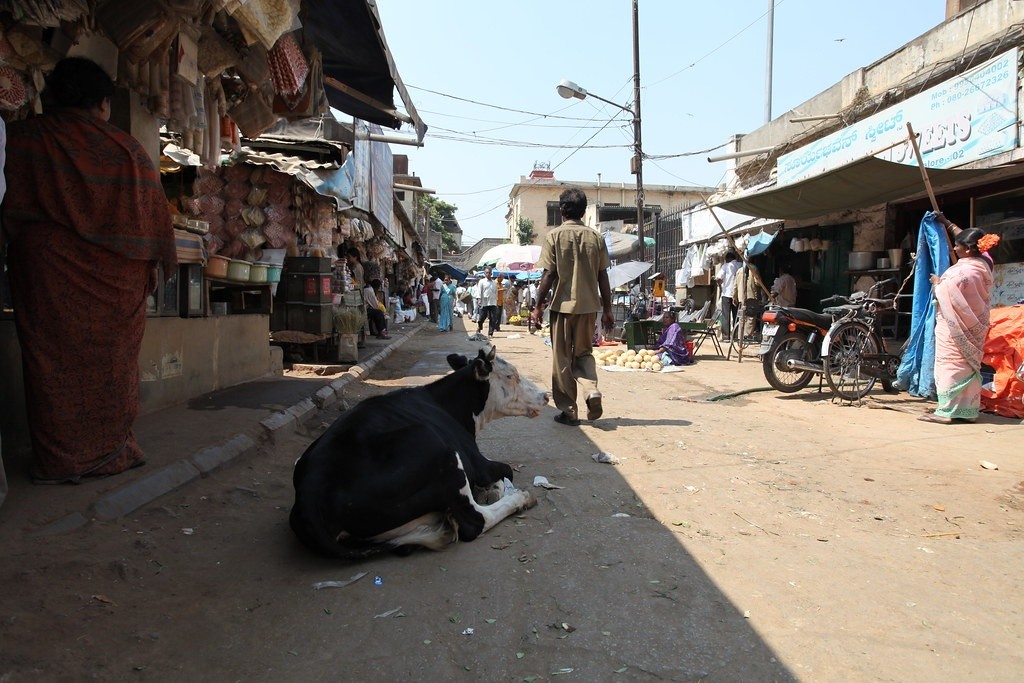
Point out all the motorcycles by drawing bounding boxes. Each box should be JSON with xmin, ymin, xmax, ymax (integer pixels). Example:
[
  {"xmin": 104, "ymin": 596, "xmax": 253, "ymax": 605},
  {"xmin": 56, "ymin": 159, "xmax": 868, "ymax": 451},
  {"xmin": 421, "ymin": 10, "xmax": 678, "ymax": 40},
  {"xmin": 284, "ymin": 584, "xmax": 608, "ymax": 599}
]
[{"xmin": 756, "ymin": 275, "xmax": 894, "ymax": 394}]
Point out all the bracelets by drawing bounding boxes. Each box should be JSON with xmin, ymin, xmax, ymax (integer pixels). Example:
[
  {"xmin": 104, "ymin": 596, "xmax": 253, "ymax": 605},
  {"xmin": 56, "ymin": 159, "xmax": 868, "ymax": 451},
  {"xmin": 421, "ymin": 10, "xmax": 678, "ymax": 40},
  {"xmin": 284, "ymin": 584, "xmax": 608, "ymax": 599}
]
[{"xmin": 947, "ymin": 223, "xmax": 956, "ymax": 233}]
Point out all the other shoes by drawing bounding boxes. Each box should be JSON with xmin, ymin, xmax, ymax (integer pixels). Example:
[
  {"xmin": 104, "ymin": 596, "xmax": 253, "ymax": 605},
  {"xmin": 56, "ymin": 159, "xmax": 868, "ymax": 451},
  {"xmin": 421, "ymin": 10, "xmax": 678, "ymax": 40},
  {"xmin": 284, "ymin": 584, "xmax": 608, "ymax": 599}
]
[
  {"xmin": 377, "ymin": 332, "xmax": 390, "ymax": 339},
  {"xmin": 719, "ymin": 338, "xmax": 729, "ymax": 343},
  {"xmin": 488, "ymin": 334, "xmax": 492, "ymax": 338},
  {"xmin": 554, "ymin": 412, "xmax": 579, "ymax": 426},
  {"xmin": 450, "ymin": 326, "xmax": 453, "ymax": 330},
  {"xmin": 440, "ymin": 329, "xmax": 445, "ymax": 333},
  {"xmin": 585, "ymin": 396, "xmax": 603, "ymax": 421}
]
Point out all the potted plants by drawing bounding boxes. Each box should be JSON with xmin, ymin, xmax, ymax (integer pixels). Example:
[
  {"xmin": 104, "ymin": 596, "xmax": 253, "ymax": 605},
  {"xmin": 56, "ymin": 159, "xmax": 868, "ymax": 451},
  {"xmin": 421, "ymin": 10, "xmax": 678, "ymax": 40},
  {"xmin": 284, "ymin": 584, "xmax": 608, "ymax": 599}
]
[{"xmin": 324, "ymin": 307, "xmax": 367, "ymax": 365}]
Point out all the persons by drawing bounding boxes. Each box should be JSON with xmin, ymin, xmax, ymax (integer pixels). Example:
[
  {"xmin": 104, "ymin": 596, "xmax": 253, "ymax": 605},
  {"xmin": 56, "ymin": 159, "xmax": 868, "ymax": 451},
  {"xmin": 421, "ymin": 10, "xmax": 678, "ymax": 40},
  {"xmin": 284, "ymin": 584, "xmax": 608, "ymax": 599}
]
[
  {"xmin": 495, "ymin": 273, "xmax": 507, "ymax": 332},
  {"xmin": 404, "ymin": 292, "xmax": 415, "ymax": 307},
  {"xmin": 364, "ymin": 279, "xmax": 391, "ymax": 339},
  {"xmin": 438, "ymin": 275, "xmax": 457, "ymax": 332},
  {"xmin": 476, "ymin": 266, "xmax": 499, "ymax": 340},
  {"xmin": 505, "ymin": 275, "xmax": 552, "ymax": 323},
  {"xmin": 420, "ymin": 274, "xmax": 440, "ymax": 321},
  {"xmin": 733, "ymin": 264, "xmax": 761, "ymax": 343},
  {"xmin": 455, "ymin": 283, "xmax": 467, "ymax": 318},
  {"xmin": 465, "ymin": 275, "xmax": 484, "ymax": 322},
  {"xmin": 652, "ymin": 311, "xmax": 690, "ymax": 366},
  {"xmin": 347, "ymin": 248, "xmax": 366, "ymax": 349},
  {"xmin": 2, "ymin": 57, "xmax": 175, "ymax": 483},
  {"xmin": 713, "ymin": 252, "xmax": 742, "ymax": 342},
  {"xmin": 768, "ymin": 265, "xmax": 797, "ymax": 306},
  {"xmin": 530, "ymin": 190, "xmax": 615, "ymax": 426},
  {"xmin": 919, "ymin": 210, "xmax": 1000, "ymax": 424}
]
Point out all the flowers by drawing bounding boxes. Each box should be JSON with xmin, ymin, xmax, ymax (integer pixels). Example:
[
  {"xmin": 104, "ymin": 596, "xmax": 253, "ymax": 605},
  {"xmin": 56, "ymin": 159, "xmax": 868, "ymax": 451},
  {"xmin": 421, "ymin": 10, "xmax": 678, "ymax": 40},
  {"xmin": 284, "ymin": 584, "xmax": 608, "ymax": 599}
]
[{"xmin": 977, "ymin": 234, "xmax": 1000, "ymax": 253}]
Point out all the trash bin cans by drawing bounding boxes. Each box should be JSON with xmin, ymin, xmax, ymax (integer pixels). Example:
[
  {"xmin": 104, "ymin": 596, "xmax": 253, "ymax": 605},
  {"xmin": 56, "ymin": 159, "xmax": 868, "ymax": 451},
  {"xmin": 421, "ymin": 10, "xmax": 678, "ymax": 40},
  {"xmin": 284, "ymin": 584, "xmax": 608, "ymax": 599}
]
[{"xmin": 625, "ymin": 322, "xmax": 648, "ymax": 354}]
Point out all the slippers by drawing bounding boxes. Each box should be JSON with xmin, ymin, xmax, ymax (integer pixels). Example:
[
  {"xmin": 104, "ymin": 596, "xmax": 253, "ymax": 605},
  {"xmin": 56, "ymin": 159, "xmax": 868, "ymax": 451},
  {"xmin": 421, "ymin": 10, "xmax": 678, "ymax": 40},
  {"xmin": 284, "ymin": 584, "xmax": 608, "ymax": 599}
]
[{"xmin": 917, "ymin": 413, "xmax": 950, "ymax": 423}]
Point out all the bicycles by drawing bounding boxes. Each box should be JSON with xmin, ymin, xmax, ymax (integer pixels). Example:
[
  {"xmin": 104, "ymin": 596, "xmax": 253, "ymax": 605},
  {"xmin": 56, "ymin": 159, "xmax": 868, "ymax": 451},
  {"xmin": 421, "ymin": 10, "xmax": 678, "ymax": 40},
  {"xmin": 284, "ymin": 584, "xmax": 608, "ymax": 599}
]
[{"xmin": 822, "ymin": 292, "xmax": 915, "ymax": 408}]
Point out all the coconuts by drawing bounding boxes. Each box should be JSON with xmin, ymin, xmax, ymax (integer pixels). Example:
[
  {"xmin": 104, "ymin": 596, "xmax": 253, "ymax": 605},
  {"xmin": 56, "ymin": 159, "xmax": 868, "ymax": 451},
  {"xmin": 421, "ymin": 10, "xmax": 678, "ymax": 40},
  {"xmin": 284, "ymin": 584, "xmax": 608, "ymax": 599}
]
[{"xmin": 593, "ymin": 349, "xmax": 661, "ymax": 371}]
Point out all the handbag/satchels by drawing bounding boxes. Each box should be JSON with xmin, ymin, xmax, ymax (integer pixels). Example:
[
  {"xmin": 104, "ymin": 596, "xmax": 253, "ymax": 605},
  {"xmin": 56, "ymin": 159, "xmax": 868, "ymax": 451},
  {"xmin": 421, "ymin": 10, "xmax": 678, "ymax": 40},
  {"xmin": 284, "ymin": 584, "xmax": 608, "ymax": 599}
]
[{"xmin": 746, "ymin": 298, "xmax": 758, "ymax": 317}]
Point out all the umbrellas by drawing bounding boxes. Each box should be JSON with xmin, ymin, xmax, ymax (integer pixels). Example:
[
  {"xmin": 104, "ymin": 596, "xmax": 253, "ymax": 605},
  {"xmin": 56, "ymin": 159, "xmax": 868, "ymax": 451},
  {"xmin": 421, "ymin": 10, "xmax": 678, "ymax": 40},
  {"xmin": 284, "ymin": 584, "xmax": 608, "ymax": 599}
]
[
  {"xmin": 478, "ymin": 243, "xmax": 520, "ymax": 277},
  {"xmin": 608, "ymin": 262, "xmax": 654, "ymax": 297},
  {"xmin": 497, "ymin": 245, "xmax": 542, "ymax": 332},
  {"xmin": 603, "ymin": 230, "xmax": 655, "ymax": 259}
]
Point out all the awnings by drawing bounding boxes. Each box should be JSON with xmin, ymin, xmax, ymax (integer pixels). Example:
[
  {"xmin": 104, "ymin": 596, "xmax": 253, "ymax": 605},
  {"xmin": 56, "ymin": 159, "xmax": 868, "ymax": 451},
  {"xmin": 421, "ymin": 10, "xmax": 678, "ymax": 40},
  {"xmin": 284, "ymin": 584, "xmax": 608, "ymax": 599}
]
[{"xmin": 698, "ymin": 122, "xmax": 1024, "ymax": 306}]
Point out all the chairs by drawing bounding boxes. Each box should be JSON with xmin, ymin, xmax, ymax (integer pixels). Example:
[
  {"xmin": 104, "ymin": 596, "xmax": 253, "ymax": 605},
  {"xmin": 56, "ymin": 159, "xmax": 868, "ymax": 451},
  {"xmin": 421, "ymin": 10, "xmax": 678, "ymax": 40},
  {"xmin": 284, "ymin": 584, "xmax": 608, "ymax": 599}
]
[{"xmin": 690, "ymin": 308, "xmax": 724, "ymax": 357}]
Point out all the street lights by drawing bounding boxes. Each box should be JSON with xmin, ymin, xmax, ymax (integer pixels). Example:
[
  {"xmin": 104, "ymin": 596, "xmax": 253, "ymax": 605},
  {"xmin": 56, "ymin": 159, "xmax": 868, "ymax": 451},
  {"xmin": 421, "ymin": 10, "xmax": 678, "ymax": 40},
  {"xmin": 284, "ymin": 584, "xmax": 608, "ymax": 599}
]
[{"xmin": 556, "ymin": 79, "xmax": 646, "ymax": 300}]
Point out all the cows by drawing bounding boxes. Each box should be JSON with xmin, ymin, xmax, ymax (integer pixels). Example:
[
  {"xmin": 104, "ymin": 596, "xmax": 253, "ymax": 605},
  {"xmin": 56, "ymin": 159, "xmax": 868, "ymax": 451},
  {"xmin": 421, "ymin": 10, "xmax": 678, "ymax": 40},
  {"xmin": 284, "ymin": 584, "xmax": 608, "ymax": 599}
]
[{"xmin": 289, "ymin": 346, "xmax": 549, "ymax": 565}]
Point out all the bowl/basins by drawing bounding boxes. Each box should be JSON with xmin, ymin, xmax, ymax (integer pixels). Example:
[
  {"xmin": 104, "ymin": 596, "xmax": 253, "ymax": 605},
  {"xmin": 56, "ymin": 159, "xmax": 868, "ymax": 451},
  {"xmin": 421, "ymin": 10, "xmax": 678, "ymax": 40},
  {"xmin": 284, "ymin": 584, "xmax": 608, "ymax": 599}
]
[{"xmin": 207, "ymin": 254, "xmax": 282, "ymax": 282}]
